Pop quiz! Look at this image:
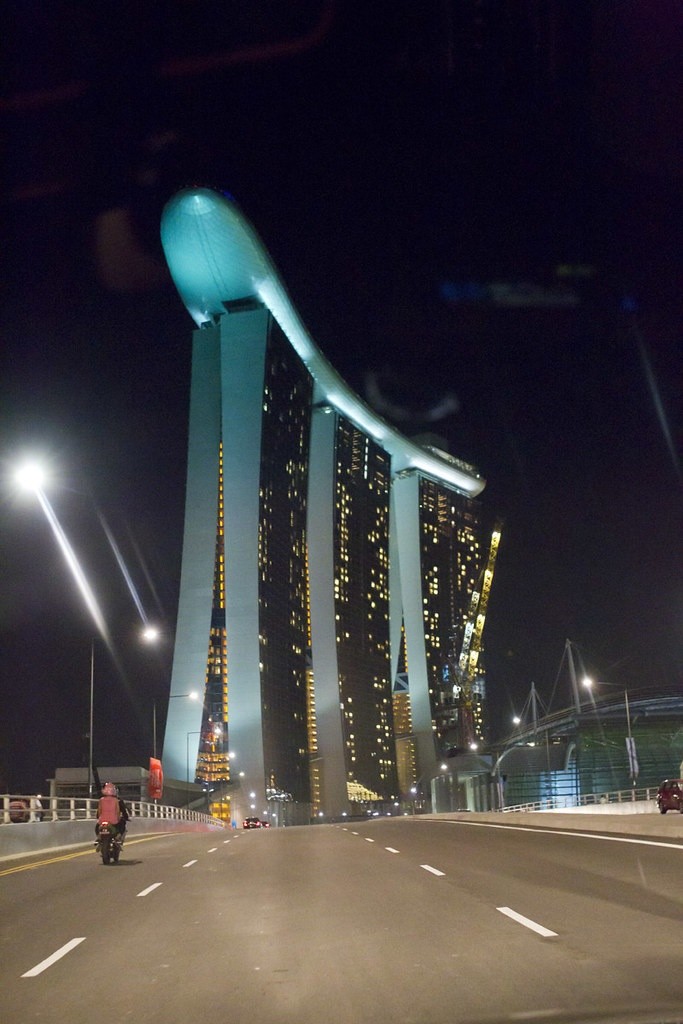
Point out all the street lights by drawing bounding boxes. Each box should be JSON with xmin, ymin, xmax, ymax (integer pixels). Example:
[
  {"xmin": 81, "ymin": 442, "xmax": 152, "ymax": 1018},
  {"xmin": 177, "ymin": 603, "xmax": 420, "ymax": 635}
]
[
  {"xmin": 186, "ymin": 729, "xmax": 221, "ymax": 809},
  {"xmin": 153, "ymin": 692, "xmax": 200, "ymax": 758},
  {"xmin": 89, "ymin": 627, "xmax": 160, "ymax": 797}
]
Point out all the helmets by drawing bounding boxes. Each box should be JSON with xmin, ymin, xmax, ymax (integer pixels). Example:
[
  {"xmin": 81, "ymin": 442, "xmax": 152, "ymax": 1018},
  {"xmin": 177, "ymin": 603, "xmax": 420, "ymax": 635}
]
[{"xmin": 103, "ymin": 782, "xmax": 116, "ymax": 795}]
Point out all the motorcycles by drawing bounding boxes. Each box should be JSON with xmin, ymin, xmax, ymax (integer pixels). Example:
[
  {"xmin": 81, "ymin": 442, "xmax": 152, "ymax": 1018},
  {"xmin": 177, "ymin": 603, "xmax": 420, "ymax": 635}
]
[{"xmin": 93, "ymin": 815, "xmax": 132, "ymax": 865}]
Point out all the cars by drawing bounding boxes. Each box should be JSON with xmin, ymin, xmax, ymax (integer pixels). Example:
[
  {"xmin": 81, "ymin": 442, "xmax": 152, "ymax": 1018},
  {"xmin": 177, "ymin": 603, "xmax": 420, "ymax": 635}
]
[
  {"xmin": 260, "ymin": 821, "xmax": 269, "ymax": 828},
  {"xmin": 657, "ymin": 778, "xmax": 683, "ymax": 814},
  {"xmin": 243, "ymin": 817, "xmax": 261, "ymax": 829}
]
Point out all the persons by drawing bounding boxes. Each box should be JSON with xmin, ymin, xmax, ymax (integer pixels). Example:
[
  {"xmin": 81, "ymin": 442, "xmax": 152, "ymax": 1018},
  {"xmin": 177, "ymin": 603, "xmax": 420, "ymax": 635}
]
[
  {"xmin": 10, "ymin": 799, "xmax": 44, "ymax": 823},
  {"xmin": 94, "ymin": 782, "xmax": 132, "ymax": 851}
]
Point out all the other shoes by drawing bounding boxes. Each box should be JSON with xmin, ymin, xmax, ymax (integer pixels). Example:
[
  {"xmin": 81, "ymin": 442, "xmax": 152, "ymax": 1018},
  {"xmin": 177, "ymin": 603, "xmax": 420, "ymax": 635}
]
[
  {"xmin": 93, "ymin": 839, "xmax": 98, "ymax": 845},
  {"xmin": 113, "ymin": 838, "xmax": 122, "ymax": 844}
]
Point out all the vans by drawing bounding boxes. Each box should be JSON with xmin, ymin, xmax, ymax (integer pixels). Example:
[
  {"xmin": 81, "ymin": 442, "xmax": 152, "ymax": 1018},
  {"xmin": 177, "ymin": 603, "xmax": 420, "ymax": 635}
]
[{"xmin": 8, "ymin": 802, "xmax": 28, "ymax": 822}]
